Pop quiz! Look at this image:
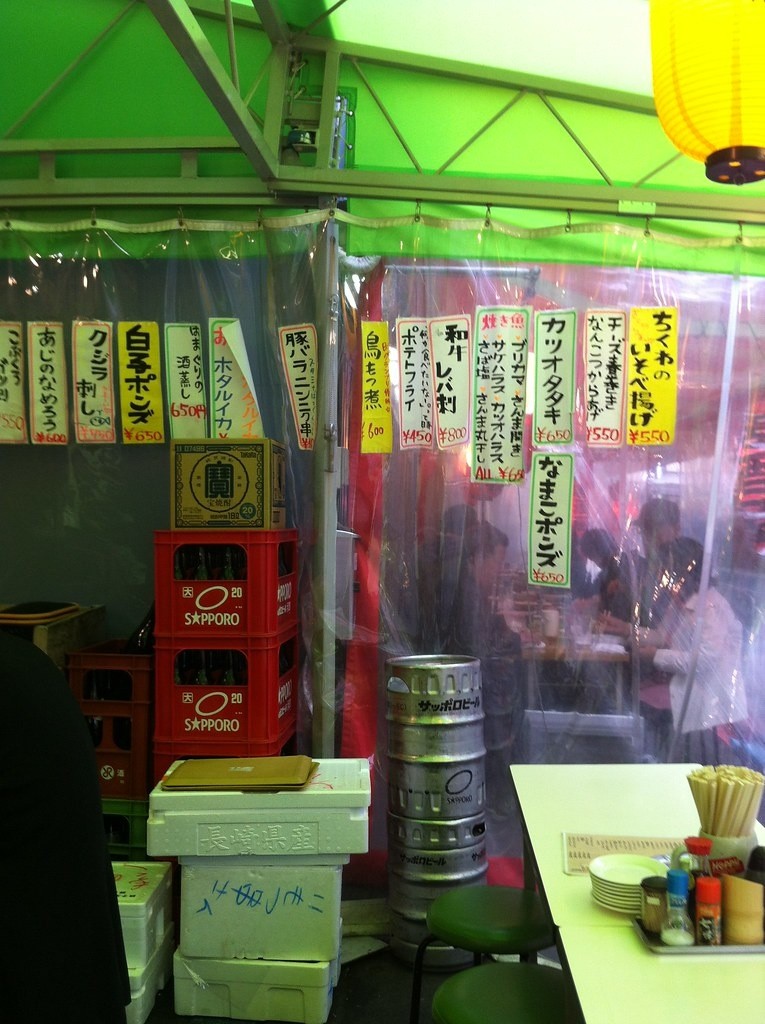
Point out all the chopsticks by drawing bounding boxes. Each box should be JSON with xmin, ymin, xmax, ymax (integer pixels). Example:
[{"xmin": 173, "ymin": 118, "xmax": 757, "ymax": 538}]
[
  {"xmin": 599, "ymin": 609, "xmax": 612, "ymax": 637},
  {"xmin": 685, "ymin": 765, "xmax": 765, "ymax": 837}
]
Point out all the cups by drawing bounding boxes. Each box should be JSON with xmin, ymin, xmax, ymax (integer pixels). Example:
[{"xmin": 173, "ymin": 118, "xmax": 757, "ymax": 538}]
[
  {"xmin": 541, "ymin": 609, "xmax": 560, "ymax": 637},
  {"xmin": 670, "ymin": 825, "xmax": 758, "ymax": 881},
  {"xmin": 721, "ymin": 873, "xmax": 764, "ymax": 946}
]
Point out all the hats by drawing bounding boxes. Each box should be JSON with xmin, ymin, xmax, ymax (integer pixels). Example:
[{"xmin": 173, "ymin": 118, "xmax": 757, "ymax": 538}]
[{"xmin": 631, "ymin": 500, "xmax": 680, "ymax": 527}]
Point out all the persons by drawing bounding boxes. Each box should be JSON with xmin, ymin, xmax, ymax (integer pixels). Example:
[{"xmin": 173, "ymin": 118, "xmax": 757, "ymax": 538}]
[
  {"xmin": 522, "ymin": 496, "xmax": 683, "ymax": 748},
  {"xmin": 412, "ymin": 506, "xmax": 479, "ymax": 645},
  {"xmin": 587, "ymin": 536, "xmax": 750, "ymax": 768},
  {"xmin": 418, "ymin": 520, "xmax": 542, "ymax": 828},
  {"xmin": 1, "ymin": 636, "xmax": 132, "ymax": 1024}
]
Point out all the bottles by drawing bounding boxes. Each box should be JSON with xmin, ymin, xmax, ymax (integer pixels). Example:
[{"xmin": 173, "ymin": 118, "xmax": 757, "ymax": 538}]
[
  {"xmin": 745, "ymin": 845, "xmax": 764, "ymax": 887},
  {"xmin": 79, "ymin": 545, "xmax": 297, "ymax": 751},
  {"xmin": 693, "ymin": 878, "xmax": 721, "ymax": 948},
  {"xmin": 671, "ymin": 837, "xmax": 717, "ymax": 920},
  {"xmin": 639, "ymin": 876, "xmax": 670, "ymax": 934},
  {"xmin": 660, "ymin": 868, "xmax": 695, "ymax": 947}
]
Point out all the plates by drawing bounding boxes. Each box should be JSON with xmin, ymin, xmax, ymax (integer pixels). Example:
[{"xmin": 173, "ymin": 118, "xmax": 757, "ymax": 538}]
[{"xmin": 588, "ymin": 854, "xmax": 670, "ymax": 915}]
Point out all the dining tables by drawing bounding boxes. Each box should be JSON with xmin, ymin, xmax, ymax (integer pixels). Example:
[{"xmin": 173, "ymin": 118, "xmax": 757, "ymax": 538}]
[
  {"xmin": 507, "ymin": 762, "xmax": 765, "ymax": 931},
  {"xmin": 486, "ymin": 564, "xmax": 644, "ymax": 763},
  {"xmin": 556, "ymin": 926, "xmax": 765, "ymax": 1024}
]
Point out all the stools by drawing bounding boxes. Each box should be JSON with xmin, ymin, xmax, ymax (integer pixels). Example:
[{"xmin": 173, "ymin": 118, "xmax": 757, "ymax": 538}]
[
  {"xmin": 409, "ymin": 885, "xmax": 557, "ymax": 1024},
  {"xmin": 661, "ymin": 724, "xmax": 721, "ymax": 766},
  {"xmin": 430, "ymin": 960, "xmax": 580, "ymax": 1024}
]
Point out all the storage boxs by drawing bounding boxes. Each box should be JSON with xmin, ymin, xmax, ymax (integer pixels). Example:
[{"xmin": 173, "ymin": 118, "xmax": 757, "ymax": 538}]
[
  {"xmin": 151, "ymin": 526, "xmax": 300, "ymax": 639},
  {"xmin": 152, "ymin": 750, "xmax": 177, "ymax": 789},
  {"xmin": 121, "ymin": 923, "xmax": 177, "ymax": 1024},
  {"xmin": 101, "ymin": 797, "xmax": 149, "ymax": 861},
  {"xmin": 153, "ymin": 638, "xmax": 299, "ymax": 758},
  {"xmin": 170, "ymin": 436, "xmax": 289, "ymax": 533},
  {"xmin": 173, "ymin": 917, "xmax": 343, "ymax": 1024},
  {"xmin": 146, "ymin": 757, "xmax": 373, "ymax": 857},
  {"xmin": 111, "ymin": 860, "xmax": 173, "ymax": 967},
  {"xmin": 177, "ymin": 854, "xmax": 351, "ymax": 961},
  {"xmin": 65, "ymin": 637, "xmax": 153, "ymax": 801}
]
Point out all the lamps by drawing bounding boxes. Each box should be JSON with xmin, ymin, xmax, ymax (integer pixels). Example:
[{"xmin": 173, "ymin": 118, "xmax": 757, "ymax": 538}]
[{"xmin": 650, "ymin": 1, "xmax": 765, "ymax": 186}]
